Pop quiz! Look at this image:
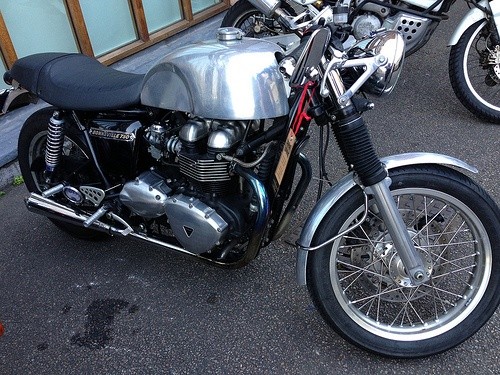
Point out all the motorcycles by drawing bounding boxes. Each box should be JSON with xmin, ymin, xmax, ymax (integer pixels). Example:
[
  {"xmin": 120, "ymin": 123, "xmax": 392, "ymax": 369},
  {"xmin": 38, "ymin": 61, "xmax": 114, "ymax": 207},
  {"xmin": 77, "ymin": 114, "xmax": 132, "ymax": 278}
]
[
  {"xmin": 2, "ymin": 15, "xmax": 499, "ymax": 359},
  {"xmin": 218, "ymin": 0, "xmax": 500, "ymax": 125}
]
